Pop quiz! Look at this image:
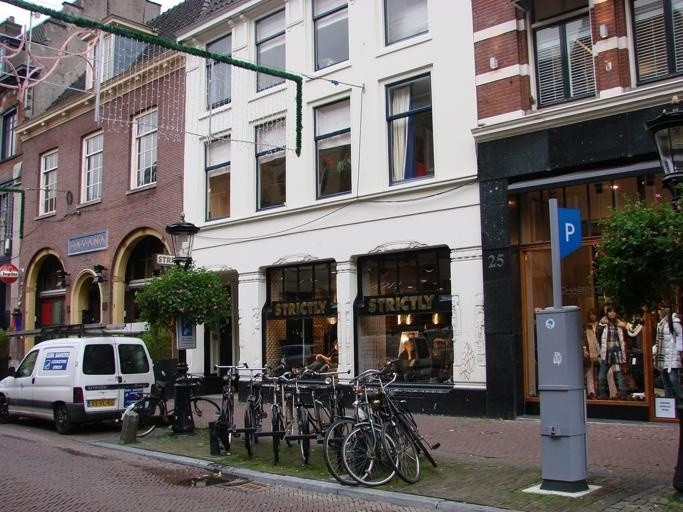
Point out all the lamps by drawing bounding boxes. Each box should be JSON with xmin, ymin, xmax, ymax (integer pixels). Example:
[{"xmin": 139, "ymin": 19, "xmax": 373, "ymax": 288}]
[
  {"xmin": 91, "ymin": 264, "xmax": 107, "ymax": 284},
  {"xmin": 54, "ymin": 270, "xmax": 70, "ymax": 288}
]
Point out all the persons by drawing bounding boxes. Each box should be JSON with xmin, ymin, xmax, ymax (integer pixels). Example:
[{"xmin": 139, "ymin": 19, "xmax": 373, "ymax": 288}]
[
  {"xmin": 580, "ymin": 300, "xmax": 682, "ymax": 406},
  {"xmin": 290, "ymin": 340, "xmax": 338, "ymax": 379}
]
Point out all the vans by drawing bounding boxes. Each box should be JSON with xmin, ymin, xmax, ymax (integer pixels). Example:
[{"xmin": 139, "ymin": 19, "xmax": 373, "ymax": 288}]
[{"xmin": 0, "ymin": 324, "xmax": 155, "ymax": 434}]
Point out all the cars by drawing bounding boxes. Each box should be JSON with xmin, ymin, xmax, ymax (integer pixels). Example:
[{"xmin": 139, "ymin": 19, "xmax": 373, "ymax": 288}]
[
  {"xmin": 386, "ymin": 327, "xmax": 453, "ymax": 382},
  {"xmin": 281, "ymin": 344, "xmax": 312, "ymax": 368}
]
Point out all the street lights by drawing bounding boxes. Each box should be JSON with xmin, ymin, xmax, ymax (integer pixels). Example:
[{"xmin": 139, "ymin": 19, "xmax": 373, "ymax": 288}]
[
  {"xmin": 643, "ymin": 97, "xmax": 683, "ymax": 492},
  {"xmin": 166, "ymin": 213, "xmax": 200, "ymax": 432}
]
